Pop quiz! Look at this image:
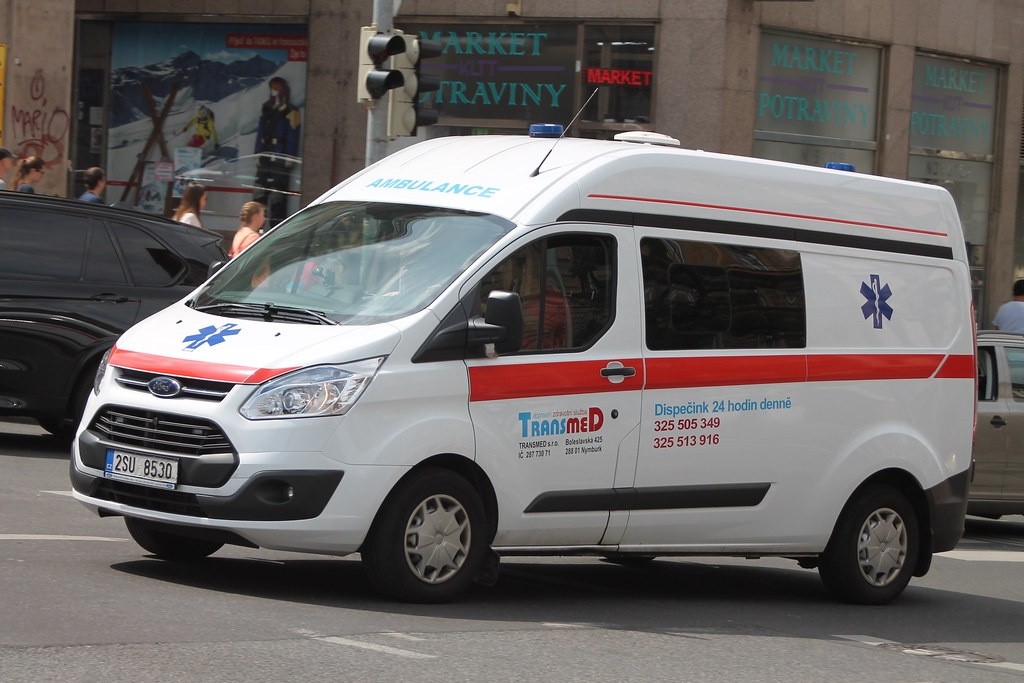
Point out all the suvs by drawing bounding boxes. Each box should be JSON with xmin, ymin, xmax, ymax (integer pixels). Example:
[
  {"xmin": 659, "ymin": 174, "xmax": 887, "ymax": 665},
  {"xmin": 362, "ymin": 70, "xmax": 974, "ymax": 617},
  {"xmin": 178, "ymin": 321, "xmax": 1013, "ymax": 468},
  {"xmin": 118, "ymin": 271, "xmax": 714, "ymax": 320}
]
[{"xmin": 1, "ymin": 188, "xmax": 237, "ymax": 435}]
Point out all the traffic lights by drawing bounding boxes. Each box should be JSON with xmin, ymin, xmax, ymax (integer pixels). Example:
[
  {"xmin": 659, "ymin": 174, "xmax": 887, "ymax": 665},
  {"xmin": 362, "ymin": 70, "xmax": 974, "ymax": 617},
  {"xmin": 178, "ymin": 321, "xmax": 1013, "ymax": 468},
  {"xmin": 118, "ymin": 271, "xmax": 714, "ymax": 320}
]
[
  {"xmin": 385, "ymin": 30, "xmax": 446, "ymax": 139},
  {"xmin": 357, "ymin": 25, "xmax": 407, "ymax": 108}
]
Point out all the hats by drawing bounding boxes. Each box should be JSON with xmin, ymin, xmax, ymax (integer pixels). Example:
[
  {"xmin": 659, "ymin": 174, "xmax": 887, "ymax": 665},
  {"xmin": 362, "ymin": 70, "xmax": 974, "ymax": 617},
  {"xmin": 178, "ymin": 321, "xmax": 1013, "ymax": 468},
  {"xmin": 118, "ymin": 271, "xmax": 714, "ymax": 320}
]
[{"xmin": 0, "ymin": 147, "xmax": 18, "ymax": 160}]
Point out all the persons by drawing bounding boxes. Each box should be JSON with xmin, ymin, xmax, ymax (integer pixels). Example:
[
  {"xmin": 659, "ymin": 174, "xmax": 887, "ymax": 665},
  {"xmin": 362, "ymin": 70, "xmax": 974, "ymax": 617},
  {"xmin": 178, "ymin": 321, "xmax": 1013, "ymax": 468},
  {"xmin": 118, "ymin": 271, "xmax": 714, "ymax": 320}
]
[
  {"xmin": 78, "ymin": 167, "xmax": 106, "ymax": 205},
  {"xmin": 992, "ymin": 279, "xmax": 1024, "ymax": 335},
  {"xmin": 0, "ymin": 148, "xmax": 17, "ymax": 190},
  {"xmin": 253, "ymin": 77, "xmax": 301, "ymax": 229},
  {"xmin": 481, "ymin": 263, "xmax": 574, "ymax": 350},
  {"xmin": 173, "ymin": 185, "xmax": 207, "ymax": 228},
  {"xmin": 182, "ymin": 105, "xmax": 219, "ymax": 151},
  {"xmin": 227, "ymin": 202, "xmax": 266, "ymax": 261},
  {"xmin": 14, "ymin": 156, "xmax": 44, "ymax": 194}
]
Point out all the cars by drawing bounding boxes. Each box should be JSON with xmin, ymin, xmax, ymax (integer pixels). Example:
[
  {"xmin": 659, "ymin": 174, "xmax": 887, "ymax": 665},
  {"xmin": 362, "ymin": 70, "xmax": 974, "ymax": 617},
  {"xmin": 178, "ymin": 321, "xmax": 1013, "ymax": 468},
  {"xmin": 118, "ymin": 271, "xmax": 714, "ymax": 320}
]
[
  {"xmin": 966, "ymin": 330, "xmax": 1024, "ymax": 517},
  {"xmin": 171, "ymin": 152, "xmax": 301, "ymax": 225}
]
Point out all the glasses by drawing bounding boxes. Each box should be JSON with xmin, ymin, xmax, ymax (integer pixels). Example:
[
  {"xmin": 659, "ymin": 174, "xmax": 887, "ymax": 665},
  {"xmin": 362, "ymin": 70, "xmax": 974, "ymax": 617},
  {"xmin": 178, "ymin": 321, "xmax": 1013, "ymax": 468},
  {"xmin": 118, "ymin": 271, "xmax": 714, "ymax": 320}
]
[{"xmin": 39, "ymin": 171, "xmax": 44, "ymax": 174}]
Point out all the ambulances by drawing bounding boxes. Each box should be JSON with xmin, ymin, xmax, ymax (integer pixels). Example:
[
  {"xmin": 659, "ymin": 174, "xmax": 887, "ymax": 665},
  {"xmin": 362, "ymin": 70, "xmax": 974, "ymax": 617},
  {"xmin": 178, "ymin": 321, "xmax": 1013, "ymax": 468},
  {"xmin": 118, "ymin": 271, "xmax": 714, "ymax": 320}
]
[{"xmin": 69, "ymin": 123, "xmax": 977, "ymax": 606}]
